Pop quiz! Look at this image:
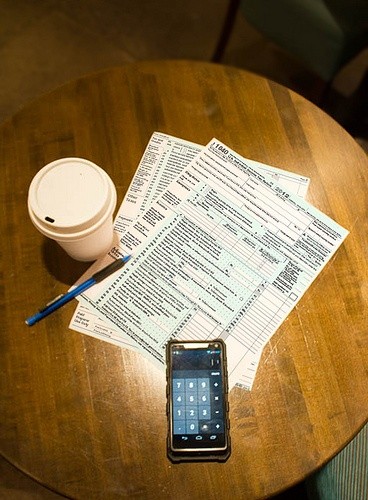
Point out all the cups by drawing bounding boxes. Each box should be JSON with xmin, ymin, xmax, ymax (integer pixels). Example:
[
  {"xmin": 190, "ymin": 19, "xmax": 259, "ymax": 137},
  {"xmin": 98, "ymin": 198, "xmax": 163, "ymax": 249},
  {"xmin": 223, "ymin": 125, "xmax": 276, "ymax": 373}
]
[{"xmin": 27, "ymin": 157, "xmax": 118, "ymax": 263}]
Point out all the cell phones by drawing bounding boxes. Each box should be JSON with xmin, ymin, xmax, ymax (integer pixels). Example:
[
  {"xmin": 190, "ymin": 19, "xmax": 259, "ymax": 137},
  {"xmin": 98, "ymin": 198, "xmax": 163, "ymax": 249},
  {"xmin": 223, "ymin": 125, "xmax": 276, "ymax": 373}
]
[{"xmin": 167, "ymin": 339, "xmax": 231, "ymax": 464}]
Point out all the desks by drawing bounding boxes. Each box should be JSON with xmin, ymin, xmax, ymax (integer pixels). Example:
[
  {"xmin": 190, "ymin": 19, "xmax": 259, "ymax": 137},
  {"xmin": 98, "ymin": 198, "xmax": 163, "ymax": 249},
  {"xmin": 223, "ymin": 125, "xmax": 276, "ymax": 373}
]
[{"xmin": 1, "ymin": 59, "xmax": 367, "ymax": 498}]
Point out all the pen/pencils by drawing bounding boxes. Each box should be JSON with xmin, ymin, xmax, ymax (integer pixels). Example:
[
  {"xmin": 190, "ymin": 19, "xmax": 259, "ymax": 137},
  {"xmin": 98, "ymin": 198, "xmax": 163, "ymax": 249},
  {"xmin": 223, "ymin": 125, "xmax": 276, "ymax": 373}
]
[{"xmin": 24, "ymin": 253, "xmax": 132, "ymax": 327}]
